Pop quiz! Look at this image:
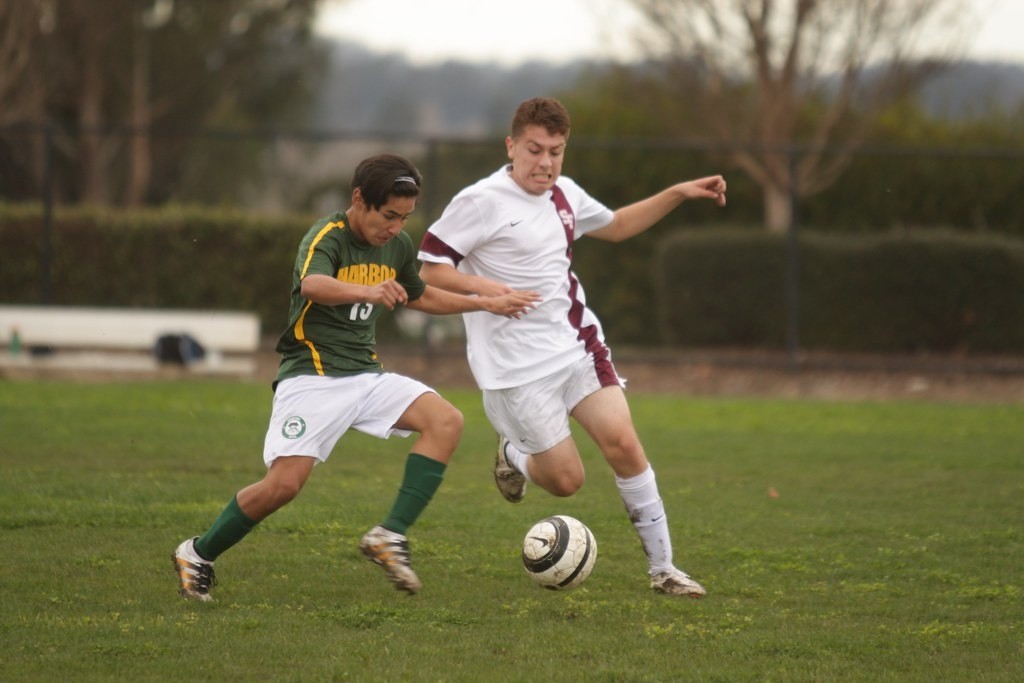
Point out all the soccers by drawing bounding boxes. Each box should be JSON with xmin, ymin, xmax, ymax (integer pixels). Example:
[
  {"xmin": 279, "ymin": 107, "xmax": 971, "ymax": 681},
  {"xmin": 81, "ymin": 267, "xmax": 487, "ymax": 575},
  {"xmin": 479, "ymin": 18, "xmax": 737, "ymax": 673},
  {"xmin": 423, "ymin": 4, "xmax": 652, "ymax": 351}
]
[{"xmin": 522, "ymin": 514, "xmax": 597, "ymax": 592}]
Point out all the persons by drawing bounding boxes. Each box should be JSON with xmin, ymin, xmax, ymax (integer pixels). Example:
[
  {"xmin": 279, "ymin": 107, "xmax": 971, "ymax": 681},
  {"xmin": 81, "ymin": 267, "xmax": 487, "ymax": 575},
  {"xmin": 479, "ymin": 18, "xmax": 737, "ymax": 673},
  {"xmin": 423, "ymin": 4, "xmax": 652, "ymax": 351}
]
[
  {"xmin": 168, "ymin": 153, "xmax": 544, "ymax": 604},
  {"xmin": 412, "ymin": 94, "xmax": 730, "ymax": 598}
]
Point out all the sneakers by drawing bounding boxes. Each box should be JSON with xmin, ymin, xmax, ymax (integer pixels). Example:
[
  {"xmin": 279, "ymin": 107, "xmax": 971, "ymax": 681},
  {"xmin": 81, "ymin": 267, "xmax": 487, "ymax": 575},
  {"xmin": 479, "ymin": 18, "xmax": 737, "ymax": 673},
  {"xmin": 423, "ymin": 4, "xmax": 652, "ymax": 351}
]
[
  {"xmin": 494, "ymin": 434, "xmax": 526, "ymax": 503},
  {"xmin": 171, "ymin": 536, "xmax": 220, "ymax": 604},
  {"xmin": 649, "ymin": 570, "xmax": 707, "ymax": 598},
  {"xmin": 358, "ymin": 525, "xmax": 423, "ymax": 594}
]
